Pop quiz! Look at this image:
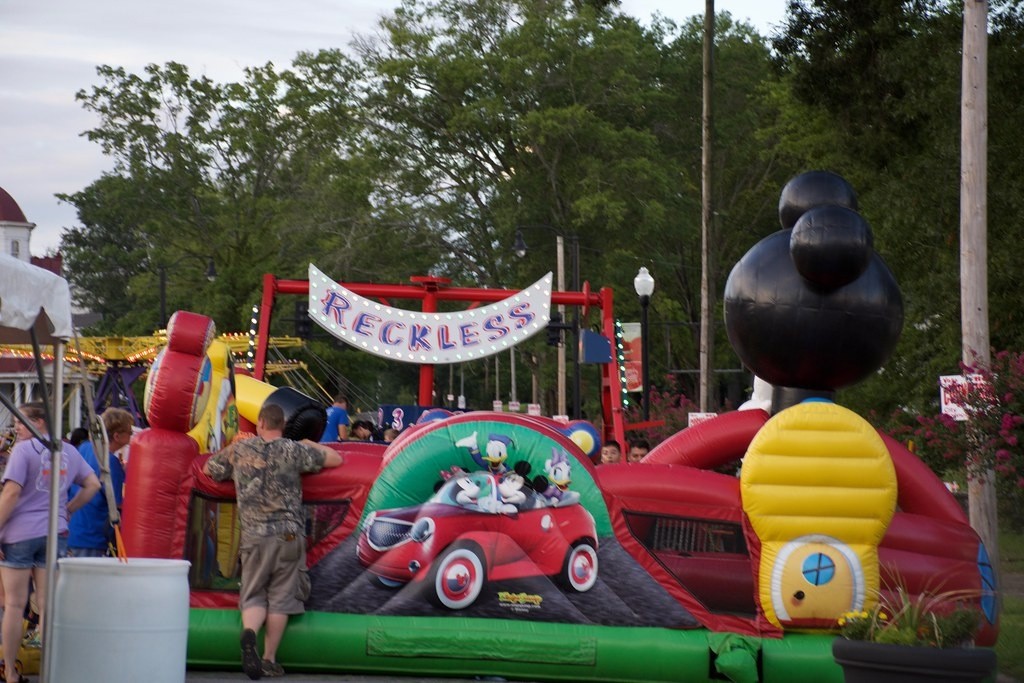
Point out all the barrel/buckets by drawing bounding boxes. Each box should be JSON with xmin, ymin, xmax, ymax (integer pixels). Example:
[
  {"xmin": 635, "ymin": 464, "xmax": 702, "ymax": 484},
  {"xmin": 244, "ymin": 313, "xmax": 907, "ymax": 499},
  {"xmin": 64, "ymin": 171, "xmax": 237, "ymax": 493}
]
[{"xmin": 48, "ymin": 557, "xmax": 192, "ymax": 683}]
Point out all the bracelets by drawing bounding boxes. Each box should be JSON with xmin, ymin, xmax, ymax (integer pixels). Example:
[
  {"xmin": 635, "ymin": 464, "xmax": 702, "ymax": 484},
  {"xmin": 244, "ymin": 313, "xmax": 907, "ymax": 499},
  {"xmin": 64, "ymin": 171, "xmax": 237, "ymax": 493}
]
[{"xmin": 65, "ymin": 506, "xmax": 73, "ymax": 516}]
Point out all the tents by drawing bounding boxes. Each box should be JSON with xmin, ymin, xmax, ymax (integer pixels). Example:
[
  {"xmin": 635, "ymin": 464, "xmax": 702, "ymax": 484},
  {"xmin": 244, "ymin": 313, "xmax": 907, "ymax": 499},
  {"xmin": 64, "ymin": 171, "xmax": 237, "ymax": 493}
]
[{"xmin": 0, "ymin": 254, "xmax": 73, "ymax": 683}]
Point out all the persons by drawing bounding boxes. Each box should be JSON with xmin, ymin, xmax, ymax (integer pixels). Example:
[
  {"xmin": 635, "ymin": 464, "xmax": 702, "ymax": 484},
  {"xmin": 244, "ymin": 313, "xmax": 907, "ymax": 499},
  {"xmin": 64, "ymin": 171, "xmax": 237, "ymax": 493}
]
[
  {"xmin": 0, "ymin": 402, "xmax": 135, "ymax": 683},
  {"xmin": 628, "ymin": 439, "xmax": 650, "ymax": 464},
  {"xmin": 352, "ymin": 419, "xmax": 399, "ymax": 443},
  {"xmin": 202, "ymin": 403, "xmax": 344, "ymax": 681},
  {"xmin": 599, "ymin": 440, "xmax": 624, "ymax": 465},
  {"xmin": 318, "ymin": 393, "xmax": 350, "ymax": 444}
]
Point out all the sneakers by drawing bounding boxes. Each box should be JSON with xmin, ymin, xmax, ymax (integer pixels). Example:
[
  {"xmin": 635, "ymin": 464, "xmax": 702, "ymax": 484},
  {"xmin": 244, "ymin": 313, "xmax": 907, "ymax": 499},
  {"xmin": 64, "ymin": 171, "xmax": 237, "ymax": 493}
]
[
  {"xmin": 260, "ymin": 660, "xmax": 285, "ymax": 677},
  {"xmin": 241, "ymin": 629, "xmax": 262, "ymax": 679}
]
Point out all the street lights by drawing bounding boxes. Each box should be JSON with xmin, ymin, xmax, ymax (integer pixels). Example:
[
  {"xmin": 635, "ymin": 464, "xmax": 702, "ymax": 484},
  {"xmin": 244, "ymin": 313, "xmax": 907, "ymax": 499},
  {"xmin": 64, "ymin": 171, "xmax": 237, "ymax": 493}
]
[
  {"xmin": 510, "ymin": 223, "xmax": 581, "ymax": 422},
  {"xmin": 157, "ymin": 254, "xmax": 218, "ymax": 330},
  {"xmin": 633, "ymin": 266, "xmax": 655, "ymax": 438}
]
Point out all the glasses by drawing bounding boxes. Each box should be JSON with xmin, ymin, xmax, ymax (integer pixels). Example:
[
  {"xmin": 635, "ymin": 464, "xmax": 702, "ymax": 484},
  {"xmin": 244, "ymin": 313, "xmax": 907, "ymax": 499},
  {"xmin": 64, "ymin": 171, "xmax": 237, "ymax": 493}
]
[{"xmin": 120, "ymin": 428, "xmax": 134, "ymax": 436}]
